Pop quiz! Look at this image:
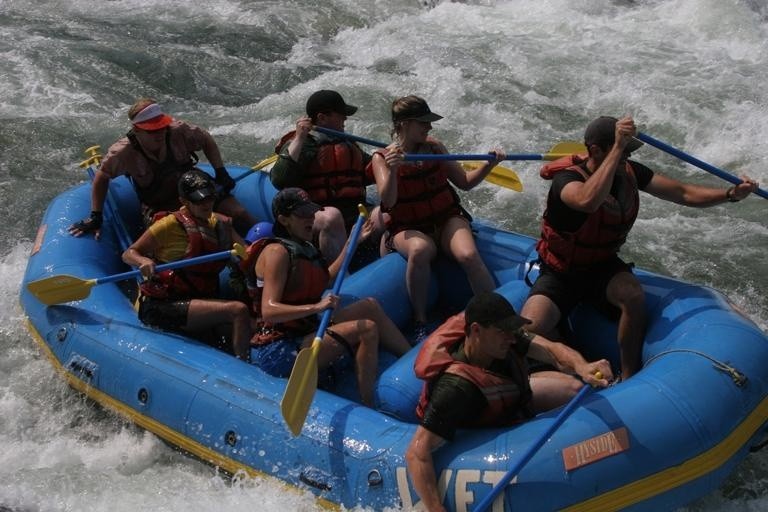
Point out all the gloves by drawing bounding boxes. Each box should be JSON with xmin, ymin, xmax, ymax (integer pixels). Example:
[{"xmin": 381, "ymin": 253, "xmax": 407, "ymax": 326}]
[
  {"xmin": 215, "ymin": 167, "xmax": 235, "ymax": 194},
  {"xmin": 73, "ymin": 210, "xmax": 103, "ymax": 232}
]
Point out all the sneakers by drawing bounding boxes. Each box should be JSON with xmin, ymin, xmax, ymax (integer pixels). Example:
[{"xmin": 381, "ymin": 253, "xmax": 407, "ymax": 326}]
[{"xmin": 410, "ymin": 321, "xmax": 428, "ymax": 346}]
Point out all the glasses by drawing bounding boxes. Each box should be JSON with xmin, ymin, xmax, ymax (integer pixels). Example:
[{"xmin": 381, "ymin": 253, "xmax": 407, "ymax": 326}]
[
  {"xmin": 477, "ymin": 322, "xmax": 525, "ymax": 338},
  {"xmin": 184, "ymin": 195, "xmax": 216, "ymax": 204},
  {"xmin": 137, "ymin": 127, "xmax": 166, "ymax": 134}
]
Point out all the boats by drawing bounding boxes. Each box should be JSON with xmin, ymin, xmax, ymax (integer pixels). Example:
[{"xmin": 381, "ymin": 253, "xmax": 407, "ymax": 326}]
[{"xmin": 18, "ymin": 158, "xmax": 767, "ymax": 512}]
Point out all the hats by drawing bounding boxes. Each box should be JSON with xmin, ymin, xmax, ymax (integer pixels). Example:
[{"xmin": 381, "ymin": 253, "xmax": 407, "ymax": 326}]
[
  {"xmin": 583, "ymin": 116, "xmax": 643, "ymax": 156},
  {"xmin": 307, "ymin": 90, "xmax": 358, "ymax": 116},
  {"xmin": 178, "ymin": 170, "xmax": 218, "ymax": 204},
  {"xmin": 272, "ymin": 187, "xmax": 322, "ymax": 218},
  {"xmin": 131, "ymin": 103, "xmax": 172, "ymax": 130},
  {"xmin": 391, "ymin": 96, "xmax": 444, "ymax": 122},
  {"xmin": 465, "ymin": 292, "xmax": 533, "ymax": 331}
]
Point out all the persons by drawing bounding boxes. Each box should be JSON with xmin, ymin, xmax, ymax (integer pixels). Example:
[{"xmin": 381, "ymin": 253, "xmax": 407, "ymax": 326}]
[
  {"xmin": 270, "ymin": 91, "xmax": 391, "ymax": 281},
  {"xmin": 406, "ymin": 292, "xmax": 612, "ymax": 512},
  {"xmin": 520, "ymin": 115, "xmax": 759, "ymax": 384},
  {"xmin": 371, "ymin": 95, "xmax": 505, "ymax": 347},
  {"xmin": 121, "ymin": 175, "xmax": 253, "ymax": 360},
  {"xmin": 246, "ymin": 187, "xmax": 411, "ymax": 409},
  {"xmin": 66, "ymin": 98, "xmax": 237, "ymax": 240}
]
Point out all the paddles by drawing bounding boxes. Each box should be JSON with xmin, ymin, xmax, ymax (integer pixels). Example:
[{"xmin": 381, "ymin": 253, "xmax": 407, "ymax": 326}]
[
  {"xmin": 78, "ymin": 155, "xmax": 144, "ymax": 312},
  {"xmin": 281, "ymin": 204, "xmax": 368, "ymax": 436},
  {"xmin": 397, "ymin": 141, "xmax": 588, "ymax": 161},
  {"xmin": 309, "ymin": 124, "xmax": 523, "ymax": 192},
  {"xmin": 27, "ymin": 243, "xmax": 249, "ymax": 305},
  {"xmin": 234, "ymin": 155, "xmax": 281, "ymax": 182}
]
